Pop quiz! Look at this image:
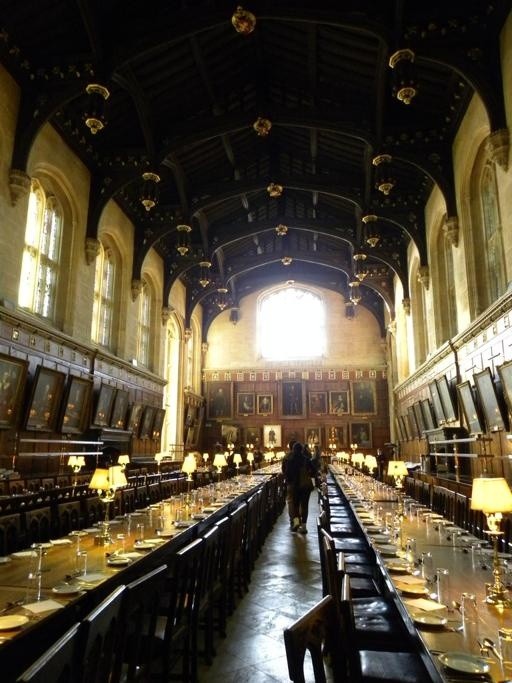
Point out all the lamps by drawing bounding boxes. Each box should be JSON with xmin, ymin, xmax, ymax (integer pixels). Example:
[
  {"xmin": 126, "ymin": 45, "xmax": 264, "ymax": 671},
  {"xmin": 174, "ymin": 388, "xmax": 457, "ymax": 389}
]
[
  {"xmin": 275, "ymin": 218, "xmax": 303, "ymax": 291},
  {"xmin": 347, "ymin": 280, "xmax": 366, "ymax": 307},
  {"xmin": 196, "ymin": 258, "xmax": 214, "ymax": 289},
  {"xmin": 137, "ymin": 168, "xmax": 166, "ymax": 212},
  {"xmin": 386, "ymin": 33, "xmax": 416, "ymax": 110},
  {"xmin": 370, "ymin": 146, "xmax": 394, "ymax": 198},
  {"xmin": 248, "ymin": 102, "xmax": 275, "ymax": 141},
  {"xmin": 215, "ymin": 280, "xmax": 231, "ymax": 312},
  {"xmin": 172, "ymin": 218, "xmax": 196, "ymax": 259},
  {"xmin": 228, "ymin": 3, "xmax": 259, "ymax": 38},
  {"xmin": 264, "ymin": 177, "xmax": 287, "ymax": 199},
  {"xmin": 358, "ymin": 206, "xmax": 384, "ymax": 250},
  {"xmin": 347, "ymin": 247, "xmax": 373, "ymax": 284},
  {"xmin": 86, "ymin": 77, "xmax": 116, "ymax": 138},
  {"xmin": 342, "ymin": 300, "xmax": 360, "ymax": 323},
  {"xmin": 227, "ymin": 305, "xmax": 243, "ymax": 330}
]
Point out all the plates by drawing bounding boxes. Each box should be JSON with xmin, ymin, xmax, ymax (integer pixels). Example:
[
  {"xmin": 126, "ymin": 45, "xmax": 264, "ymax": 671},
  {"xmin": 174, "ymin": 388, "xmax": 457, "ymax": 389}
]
[
  {"xmin": 0, "ymin": 499, "xmax": 227, "ymax": 631},
  {"xmin": 330, "ymin": 462, "xmax": 490, "ymax": 676}
]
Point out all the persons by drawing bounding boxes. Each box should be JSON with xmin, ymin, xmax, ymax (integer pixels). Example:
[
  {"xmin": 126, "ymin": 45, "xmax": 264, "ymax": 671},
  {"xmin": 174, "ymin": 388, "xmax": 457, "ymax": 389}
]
[
  {"xmin": 376, "ymin": 448, "xmax": 385, "ymax": 467},
  {"xmin": 282, "ymin": 439, "xmax": 321, "ymax": 534}
]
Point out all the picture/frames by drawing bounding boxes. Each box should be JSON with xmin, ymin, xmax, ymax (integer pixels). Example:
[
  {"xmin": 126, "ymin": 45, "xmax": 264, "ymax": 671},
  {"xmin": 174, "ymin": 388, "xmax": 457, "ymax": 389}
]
[
  {"xmin": 181, "ymin": 369, "xmax": 380, "ymax": 452},
  {"xmin": 1, "ymin": 350, "xmax": 167, "ymax": 444},
  {"xmin": 387, "ymin": 358, "xmax": 512, "ymax": 447}
]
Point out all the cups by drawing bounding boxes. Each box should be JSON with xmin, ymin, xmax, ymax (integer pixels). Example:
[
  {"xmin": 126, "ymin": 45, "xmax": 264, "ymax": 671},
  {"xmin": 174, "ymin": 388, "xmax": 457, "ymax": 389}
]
[
  {"xmin": 366, "ymin": 496, "xmax": 512, "ymax": 672},
  {"xmin": 26, "ymin": 474, "xmax": 252, "ymax": 601}
]
